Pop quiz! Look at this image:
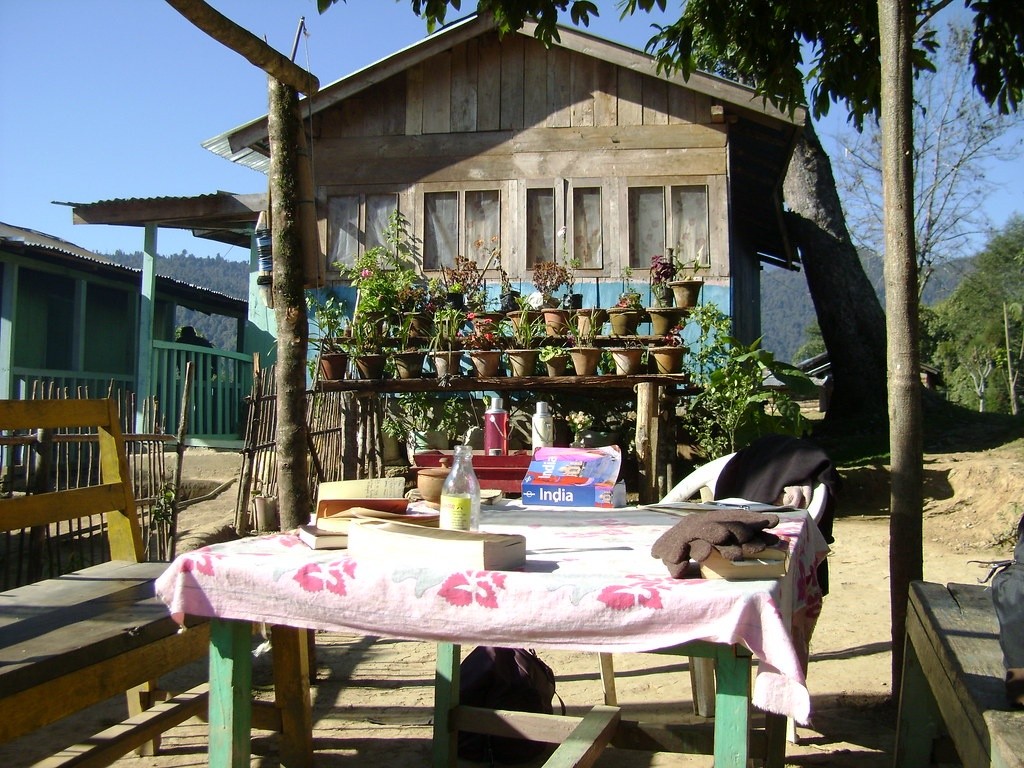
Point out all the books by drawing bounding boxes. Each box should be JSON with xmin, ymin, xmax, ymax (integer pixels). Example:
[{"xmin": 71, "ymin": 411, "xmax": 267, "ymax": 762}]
[
  {"xmin": 297, "ymin": 476, "xmax": 788, "ymax": 581},
  {"xmin": 520, "ymin": 445, "xmax": 628, "ymax": 508}
]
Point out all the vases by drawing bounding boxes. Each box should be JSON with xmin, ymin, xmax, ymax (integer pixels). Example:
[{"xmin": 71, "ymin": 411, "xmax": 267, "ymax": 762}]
[
  {"xmin": 650, "ymin": 284, "xmax": 673, "ymax": 308},
  {"xmin": 467, "ymin": 351, "xmax": 505, "ymax": 377},
  {"xmin": 569, "ymin": 435, "xmax": 586, "ymax": 450},
  {"xmin": 362, "ymin": 311, "xmax": 387, "ymax": 345}
]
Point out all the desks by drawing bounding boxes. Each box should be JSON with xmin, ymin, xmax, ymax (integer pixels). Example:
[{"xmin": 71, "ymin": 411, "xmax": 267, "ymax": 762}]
[{"xmin": 152, "ymin": 497, "xmax": 832, "ymax": 768}]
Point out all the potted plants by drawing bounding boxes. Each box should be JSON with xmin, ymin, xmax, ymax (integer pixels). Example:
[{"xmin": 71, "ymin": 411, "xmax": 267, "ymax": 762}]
[{"xmin": 313, "ymin": 206, "xmax": 754, "ymax": 502}]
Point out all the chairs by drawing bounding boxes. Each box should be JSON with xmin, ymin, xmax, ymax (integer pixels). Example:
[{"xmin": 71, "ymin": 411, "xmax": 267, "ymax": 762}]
[{"xmin": 598, "ymin": 451, "xmax": 827, "ymax": 719}]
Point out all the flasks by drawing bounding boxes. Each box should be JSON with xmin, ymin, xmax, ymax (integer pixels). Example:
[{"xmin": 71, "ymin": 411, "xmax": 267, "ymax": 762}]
[
  {"xmin": 484, "ymin": 397, "xmax": 515, "ymax": 456},
  {"xmin": 530, "ymin": 402, "xmax": 554, "ymax": 457}
]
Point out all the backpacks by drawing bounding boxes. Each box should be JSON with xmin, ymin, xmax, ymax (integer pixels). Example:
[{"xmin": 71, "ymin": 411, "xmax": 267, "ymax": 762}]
[
  {"xmin": 451, "ymin": 644, "xmax": 565, "ymax": 752},
  {"xmin": 965, "ymin": 520, "xmax": 1024, "ymax": 670}
]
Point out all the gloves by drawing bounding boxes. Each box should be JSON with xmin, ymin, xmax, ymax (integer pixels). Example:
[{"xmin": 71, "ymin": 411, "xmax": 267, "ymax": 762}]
[{"xmin": 651, "ymin": 511, "xmax": 781, "ymax": 580}]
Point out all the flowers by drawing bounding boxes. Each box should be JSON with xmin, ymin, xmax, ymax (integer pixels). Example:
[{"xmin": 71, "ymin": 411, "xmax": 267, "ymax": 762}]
[
  {"xmin": 453, "ymin": 307, "xmax": 503, "ymax": 351},
  {"xmin": 565, "ymin": 407, "xmax": 595, "ymax": 438},
  {"xmin": 647, "ymin": 253, "xmax": 701, "ymax": 304},
  {"xmin": 329, "ymin": 244, "xmax": 403, "ymax": 311}
]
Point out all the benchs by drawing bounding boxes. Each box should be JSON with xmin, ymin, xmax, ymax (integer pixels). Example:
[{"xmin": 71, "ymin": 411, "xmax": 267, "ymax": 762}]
[
  {"xmin": 1, "ymin": 398, "xmax": 282, "ymax": 768},
  {"xmin": 893, "ymin": 580, "xmax": 1024, "ymax": 768}
]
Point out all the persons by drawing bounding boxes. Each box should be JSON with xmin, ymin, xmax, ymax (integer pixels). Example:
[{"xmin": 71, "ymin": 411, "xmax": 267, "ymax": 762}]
[{"xmin": 175, "ymin": 325, "xmax": 219, "ymax": 411}]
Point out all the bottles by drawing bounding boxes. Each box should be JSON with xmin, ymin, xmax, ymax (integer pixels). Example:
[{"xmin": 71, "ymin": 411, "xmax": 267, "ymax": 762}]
[{"xmin": 438, "ymin": 444, "xmax": 481, "ymax": 532}]
